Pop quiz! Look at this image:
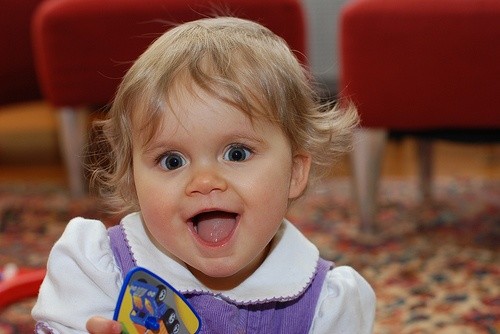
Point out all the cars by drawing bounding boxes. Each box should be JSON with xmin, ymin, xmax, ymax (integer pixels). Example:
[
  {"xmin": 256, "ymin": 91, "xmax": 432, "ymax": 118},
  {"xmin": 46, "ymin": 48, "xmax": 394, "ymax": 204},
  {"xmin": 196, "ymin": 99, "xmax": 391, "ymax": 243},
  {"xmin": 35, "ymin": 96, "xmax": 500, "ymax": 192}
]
[{"xmin": 129, "ymin": 279, "xmax": 180, "ymax": 334}]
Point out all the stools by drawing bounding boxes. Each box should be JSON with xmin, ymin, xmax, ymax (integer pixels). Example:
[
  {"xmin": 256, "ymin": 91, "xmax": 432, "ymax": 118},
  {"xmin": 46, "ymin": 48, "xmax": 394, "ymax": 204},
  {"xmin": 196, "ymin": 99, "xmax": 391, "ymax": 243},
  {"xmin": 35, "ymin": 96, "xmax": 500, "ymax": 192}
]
[
  {"xmin": 33, "ymin": 1, "xmax": 310, "ymax": 199},
  {"xmin": 336, "ymin": 2, "xmax": 499, "ymax": 233}
]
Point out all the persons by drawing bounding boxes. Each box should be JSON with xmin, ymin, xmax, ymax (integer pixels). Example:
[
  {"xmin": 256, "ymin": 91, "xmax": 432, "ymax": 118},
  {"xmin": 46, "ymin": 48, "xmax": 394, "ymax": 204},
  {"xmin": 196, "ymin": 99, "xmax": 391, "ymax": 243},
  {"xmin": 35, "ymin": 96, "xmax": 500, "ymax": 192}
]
[{"xmin": 30, "ymin": 16, "xmax": 377, "ymax": 334}]
